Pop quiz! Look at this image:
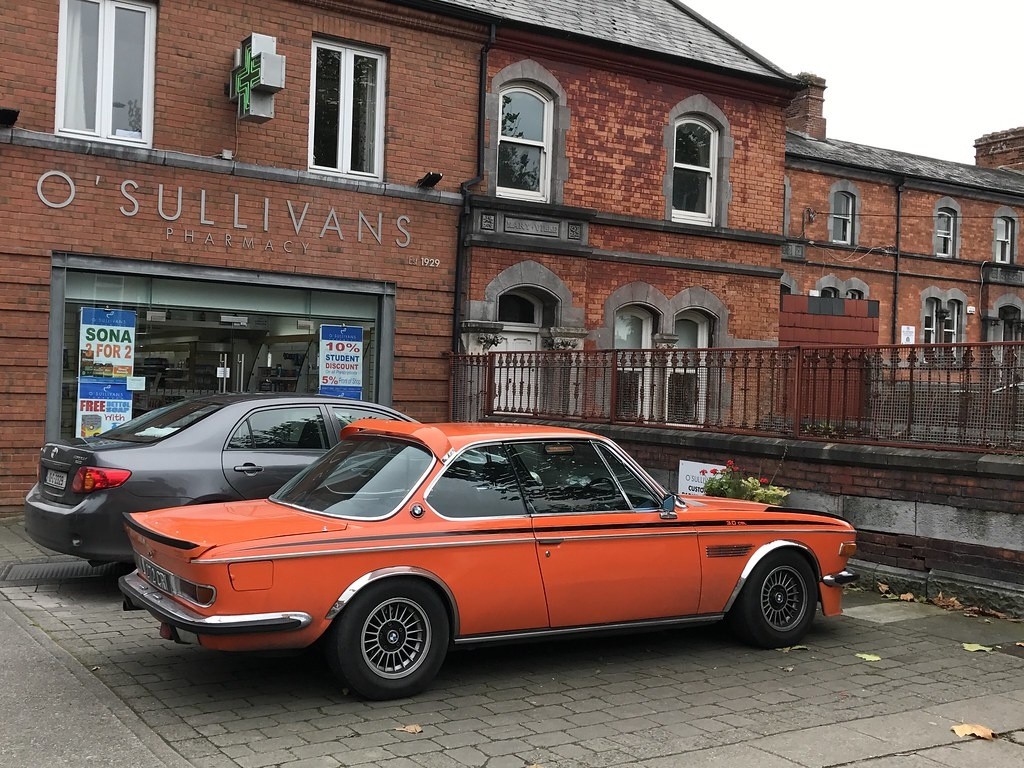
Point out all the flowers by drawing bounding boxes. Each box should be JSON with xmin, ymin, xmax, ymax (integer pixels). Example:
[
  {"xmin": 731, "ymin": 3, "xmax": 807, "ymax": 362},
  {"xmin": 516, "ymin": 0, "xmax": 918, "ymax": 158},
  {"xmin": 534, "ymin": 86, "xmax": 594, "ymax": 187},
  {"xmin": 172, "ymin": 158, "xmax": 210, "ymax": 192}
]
[{"xmin": 699, "ymin": 459, "xmax": 792, "ymax": 505}]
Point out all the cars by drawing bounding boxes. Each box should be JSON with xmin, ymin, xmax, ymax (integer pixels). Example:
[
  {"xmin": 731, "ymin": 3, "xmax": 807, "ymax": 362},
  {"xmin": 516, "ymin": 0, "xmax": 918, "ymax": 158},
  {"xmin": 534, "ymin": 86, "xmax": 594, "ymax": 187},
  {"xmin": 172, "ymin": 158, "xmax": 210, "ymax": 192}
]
[
  {"xmin": 25, "ymin": 394, "xmax": 425, "ymax": 567},
  {"xmin": 119, "ymin": 420, "xmax": 858, "ymax": 701}
]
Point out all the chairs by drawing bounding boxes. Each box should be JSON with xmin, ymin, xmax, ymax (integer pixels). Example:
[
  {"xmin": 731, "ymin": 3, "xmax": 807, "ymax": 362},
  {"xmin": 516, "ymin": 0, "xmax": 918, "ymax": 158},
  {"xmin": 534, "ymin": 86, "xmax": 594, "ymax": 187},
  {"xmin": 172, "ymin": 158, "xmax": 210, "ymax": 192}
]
[
  {"xmin": 484, "ymin": 462, "xmax": 523, "ymax": 513},
  {"xmin": 448, "ymin": 461, "xmax": 483, "ymax": 510},
  {"xmin": 297, "ymin": 419, "xmax": 321, "ymax": 449}
]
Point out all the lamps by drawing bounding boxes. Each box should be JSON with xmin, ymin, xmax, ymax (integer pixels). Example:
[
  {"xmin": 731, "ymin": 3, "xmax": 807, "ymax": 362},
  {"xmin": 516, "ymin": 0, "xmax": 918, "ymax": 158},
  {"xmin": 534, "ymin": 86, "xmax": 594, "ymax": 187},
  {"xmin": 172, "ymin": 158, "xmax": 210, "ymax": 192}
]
[
  {"xmin": 0, "ymin": 107, "xmax": 20, "ymax": 129},
  {"xmin": 419, "ymin": 171, "xmax": 443, "ymax": 189}
]
